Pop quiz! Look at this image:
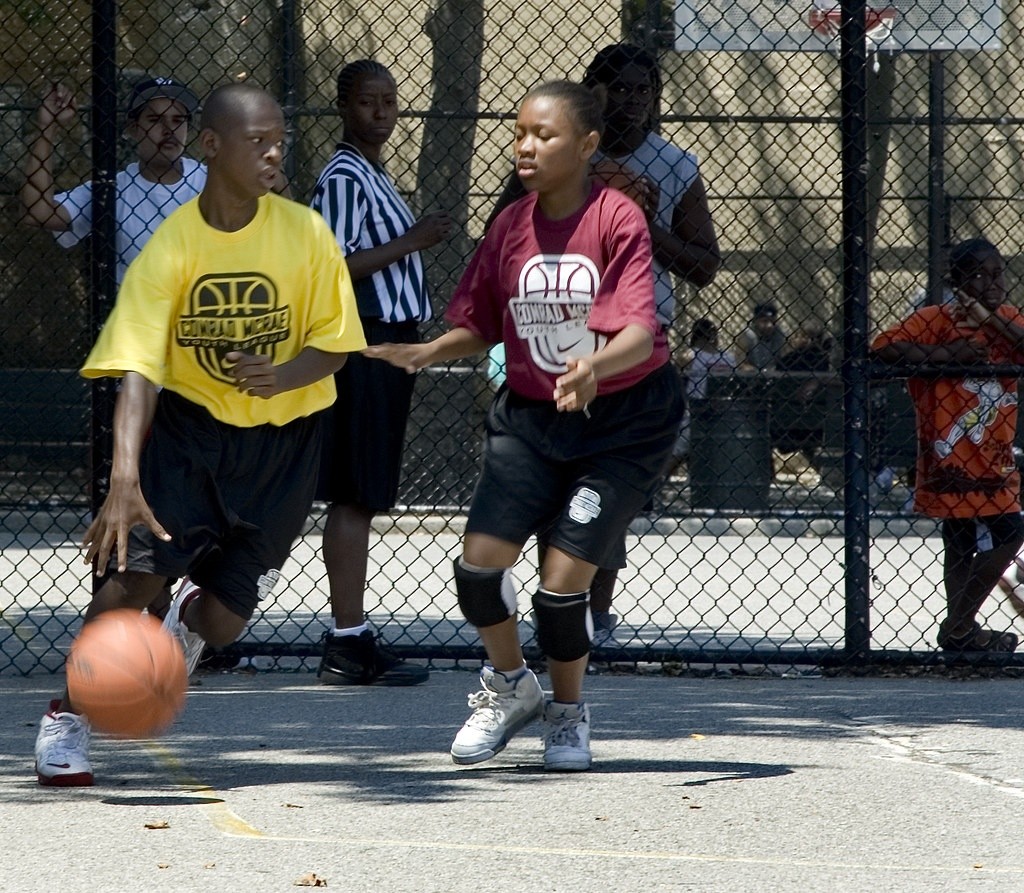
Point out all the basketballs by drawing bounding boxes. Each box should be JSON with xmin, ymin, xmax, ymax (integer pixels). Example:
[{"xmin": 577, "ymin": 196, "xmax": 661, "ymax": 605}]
[{"xmin": 65, "ymin": 607, "xmax": 188, "ymax": 736}]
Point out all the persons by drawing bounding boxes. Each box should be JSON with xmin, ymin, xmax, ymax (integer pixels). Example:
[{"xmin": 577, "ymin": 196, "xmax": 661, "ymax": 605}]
[
  {"xmin": 483, "ymin": 44, "xmax": 721, "ymax": 653},
  {"xmin": 357, "ymin": 80, "xmax": 684, "ymax": 778},
  {"xmin": 663, "ymin": 315, "xmax": 737, "ymax": 479},
  {"xmin": 868, "ymin": 239, "xmax": 1023, "ymax": 653},
  {"xmin": 34, "ymin": 83, "xmax": 367, "ymax": 789},
  {"xmin": 312, "ymin": 58, "xmax": 455, "ymax": 688},
  {"xmin": 742, "ymin": 306, "xmax": 833, "ymax": 469},
  {"xmin": 20, "ymin": 76, "xmax": 255, "ymax": 673}
]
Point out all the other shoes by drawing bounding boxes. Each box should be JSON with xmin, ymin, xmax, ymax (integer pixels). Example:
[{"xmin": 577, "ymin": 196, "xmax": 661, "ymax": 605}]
[{"xmin": 587, "ymin": 614, "xmax": 635, "ymax": 675}]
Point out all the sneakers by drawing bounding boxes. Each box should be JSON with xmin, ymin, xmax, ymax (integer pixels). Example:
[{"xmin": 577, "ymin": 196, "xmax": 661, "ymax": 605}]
[
  {"xmin": 450, "ymin": 666, "xmax": 545, "ymax": 765},
  {"xmin": 161, "ymin": 575, "xmax": 206, "ymax": 679},
  {"xmin": 542, "ymin": 699, "xmax": 592, "ymax": 771},
  {"xmin": 35, "ymin": 699, "xmax": 95, "ymax": 786},
  {"xmin": 316, "ymin": 629, "xmax": 429, "ymax": 686}
]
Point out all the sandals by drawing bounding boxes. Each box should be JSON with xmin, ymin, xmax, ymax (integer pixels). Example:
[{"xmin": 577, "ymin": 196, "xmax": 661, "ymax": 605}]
[{"xmin": 937, "ymin": 619, "xmax": 1018, "ymax": 653}]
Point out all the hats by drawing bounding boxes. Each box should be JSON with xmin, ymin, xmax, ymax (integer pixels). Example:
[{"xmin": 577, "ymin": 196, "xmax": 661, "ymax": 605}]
[{"xmin": 129, "ymin": 77, "xmax": 200, "ymax": 118}]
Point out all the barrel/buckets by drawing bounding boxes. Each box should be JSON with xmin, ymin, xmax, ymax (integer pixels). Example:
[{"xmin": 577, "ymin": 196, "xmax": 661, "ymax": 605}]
[{"xmin": 687, "ymin": 395, "xmax": 773, "ymax": 512}]
